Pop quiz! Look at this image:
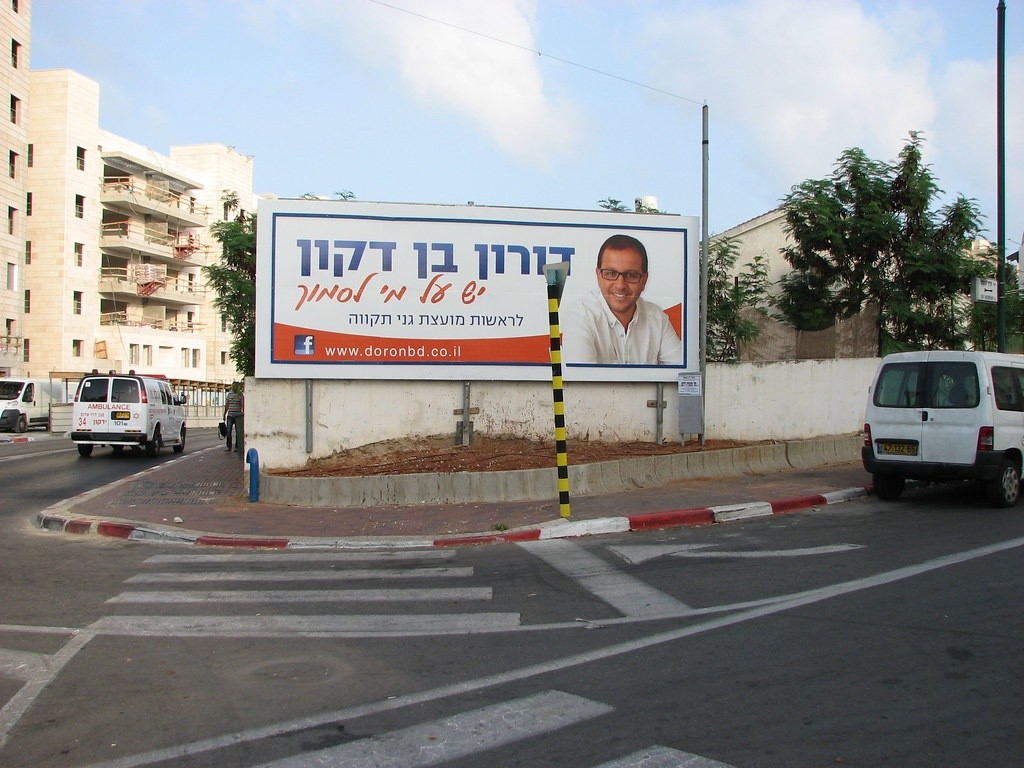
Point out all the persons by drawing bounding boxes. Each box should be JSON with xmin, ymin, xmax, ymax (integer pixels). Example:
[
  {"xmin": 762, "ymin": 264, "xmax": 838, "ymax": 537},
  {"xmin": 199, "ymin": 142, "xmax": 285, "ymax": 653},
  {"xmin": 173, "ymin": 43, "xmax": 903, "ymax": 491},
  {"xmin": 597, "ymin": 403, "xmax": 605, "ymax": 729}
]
[
  {"xmin": 224, "ymin": 381, "xmax": 244, "ymax": 452},
  {"xmin": 562, "ymin": 235, "xmax": 683, "ymax": 365}
]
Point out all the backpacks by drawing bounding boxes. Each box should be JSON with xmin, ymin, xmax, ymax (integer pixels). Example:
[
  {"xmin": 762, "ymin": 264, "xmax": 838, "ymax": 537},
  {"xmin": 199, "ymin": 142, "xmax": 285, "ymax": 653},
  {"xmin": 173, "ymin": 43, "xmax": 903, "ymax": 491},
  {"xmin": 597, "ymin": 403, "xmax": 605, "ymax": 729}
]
[{"xmin": 217, "ymin": 421, "xmax": 227, "ymax": 441}]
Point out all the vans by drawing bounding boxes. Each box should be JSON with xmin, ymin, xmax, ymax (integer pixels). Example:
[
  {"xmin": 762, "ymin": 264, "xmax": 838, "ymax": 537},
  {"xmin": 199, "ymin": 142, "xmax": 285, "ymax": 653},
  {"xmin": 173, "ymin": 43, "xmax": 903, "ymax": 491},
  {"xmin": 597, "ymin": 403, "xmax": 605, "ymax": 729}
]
[{"xmin": 861, "ymin": 349, "xmax": 1024, "ymax": 509}]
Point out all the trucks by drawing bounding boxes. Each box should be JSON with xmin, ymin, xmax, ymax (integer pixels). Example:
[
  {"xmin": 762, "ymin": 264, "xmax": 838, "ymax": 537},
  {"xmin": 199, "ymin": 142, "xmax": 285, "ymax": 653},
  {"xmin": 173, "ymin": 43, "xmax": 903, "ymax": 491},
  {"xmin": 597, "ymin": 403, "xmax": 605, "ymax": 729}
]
[{"xmin": 0, "ymin": 376, "xmax": 66, "ymax": 434}]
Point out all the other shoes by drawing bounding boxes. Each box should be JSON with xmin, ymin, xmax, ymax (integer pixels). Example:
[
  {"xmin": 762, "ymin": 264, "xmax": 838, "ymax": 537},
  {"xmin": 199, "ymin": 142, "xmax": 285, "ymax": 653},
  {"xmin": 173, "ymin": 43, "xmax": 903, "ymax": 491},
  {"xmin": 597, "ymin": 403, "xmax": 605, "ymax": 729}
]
[
  {"xmin": 225, "ymin": 447, "xmax": 231, "ymax": 452},
  {"xmin": 234, "ymin": 447, "xmax": 239, "ymax": 452}
]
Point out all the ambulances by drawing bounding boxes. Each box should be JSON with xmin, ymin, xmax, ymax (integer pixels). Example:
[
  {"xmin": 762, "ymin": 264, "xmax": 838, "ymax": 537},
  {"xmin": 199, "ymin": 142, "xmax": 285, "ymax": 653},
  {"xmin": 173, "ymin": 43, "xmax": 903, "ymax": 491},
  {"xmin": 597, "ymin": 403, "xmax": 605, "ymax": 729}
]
[{"xmin": 70, "ymin": 369, "xmax": 188, "ymax": 460}]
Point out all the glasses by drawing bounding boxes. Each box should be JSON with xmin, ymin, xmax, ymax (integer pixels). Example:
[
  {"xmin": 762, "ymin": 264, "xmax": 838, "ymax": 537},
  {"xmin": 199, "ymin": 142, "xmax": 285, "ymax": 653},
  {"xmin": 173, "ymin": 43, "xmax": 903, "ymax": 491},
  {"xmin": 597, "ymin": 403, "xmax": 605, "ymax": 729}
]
[{"xmin": 600, "ymin": 268, "xmax": 645, "ymax": 283}]
